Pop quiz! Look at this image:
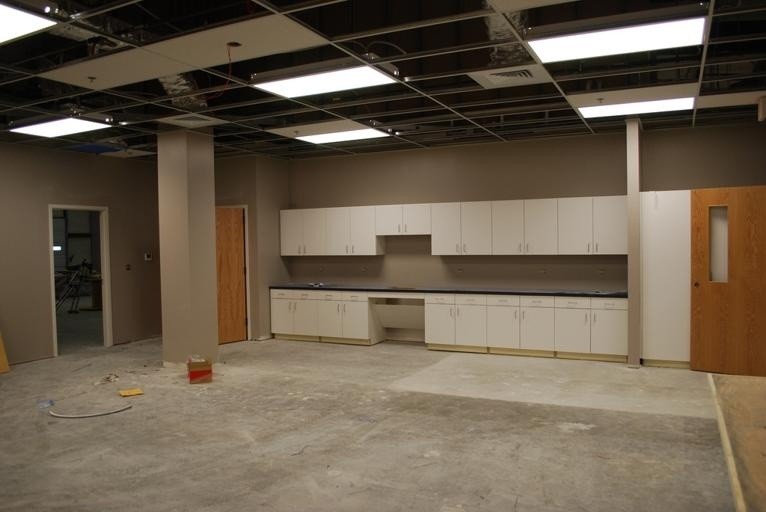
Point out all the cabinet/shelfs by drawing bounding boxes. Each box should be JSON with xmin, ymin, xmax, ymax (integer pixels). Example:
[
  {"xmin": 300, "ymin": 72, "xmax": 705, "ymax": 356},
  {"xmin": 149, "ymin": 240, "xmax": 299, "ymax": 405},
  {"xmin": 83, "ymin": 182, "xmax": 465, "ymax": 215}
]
[
  {"xmin": 491, "ymin": 199, "xmax": 558, "ymax": 256},
  {"xmin": 318, "ymin": 290, "xmax": 385, "ymax": 339},
  {"xmin": 555, "ymin": 296, "xmax": 628, "ymax": 356},
  {"xmin": 430, "ymin": 202, "xmax": 492, "ymax": 256},
  {"xmin": 279, "ymin": 208, "xmax": 326, "ymax": 257},
  {"xmin": 486, "ymin": 294, "xmax": 555, "ymax": 352},
  {"xmin": 327, "ymin": 206, "xmax": 385, "ymax": 257},
  {"xmin": 375, "ymin": 204, "xmax": 431, "ymax": 237},
  {"xmin": 423, "ymin": 293, "xmax": 486, "ymax": 349},
  {"xmin": 270, "ymin": 288, "xmax": 319, "ymax": 337},
  {"xmin": 557, "ymin": 195, "xmax": 627, "ymax": 257}
]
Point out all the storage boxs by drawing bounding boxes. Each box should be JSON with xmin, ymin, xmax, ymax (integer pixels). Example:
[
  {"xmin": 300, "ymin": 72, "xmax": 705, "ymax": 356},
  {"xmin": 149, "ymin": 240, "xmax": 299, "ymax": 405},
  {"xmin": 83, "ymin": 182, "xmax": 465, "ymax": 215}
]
[{"xmin": 188, "ymin": 361, "xmax": 212, "ymax": 384}]
